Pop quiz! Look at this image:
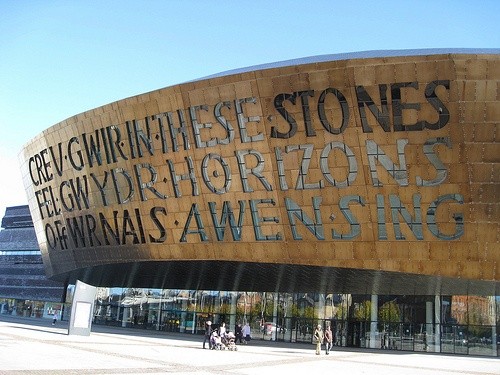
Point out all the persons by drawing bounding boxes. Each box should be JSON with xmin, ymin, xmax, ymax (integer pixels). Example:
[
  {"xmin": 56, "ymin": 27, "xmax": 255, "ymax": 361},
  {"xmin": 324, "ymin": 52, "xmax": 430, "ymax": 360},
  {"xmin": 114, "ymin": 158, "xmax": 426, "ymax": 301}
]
[
  {"xmin": 234, "ymin": 322, "xmax": 251, "ymax": 345},
  {"xmin": 203, "ymin": 321, "xmax": 213, "ymax": 349},
  {"xmin": 299, "ymin": 320, "xmax": 311, "ymax": 338},
  {"xmin": 315, "ymin": 323, "xmax": 322, "ymax": 355},
  {"xmin": 324, "ymin": 324, "xmax": 332, "ymax": 355},
  {"xmin": 51, "ymin": 309, "xmax": 58, "ymax": 326},
  {"xmin": 279, "ymin": 324, "xmax": 287, "ymax": 335},
  {"xmin": 219, "ymin": 322, "xmax": 226, "ymax": 344}
]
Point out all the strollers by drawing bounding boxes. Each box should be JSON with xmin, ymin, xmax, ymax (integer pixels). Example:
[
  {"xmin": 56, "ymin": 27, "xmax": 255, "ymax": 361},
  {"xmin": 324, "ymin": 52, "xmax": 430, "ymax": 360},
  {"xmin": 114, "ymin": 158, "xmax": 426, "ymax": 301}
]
[
  {"xmin": 208, "ymin": 328, "xmax": 225, "ymax": 351},
  {"xmin": 221, "ymin": 330, "xmax": 238, "ymax": 351}
]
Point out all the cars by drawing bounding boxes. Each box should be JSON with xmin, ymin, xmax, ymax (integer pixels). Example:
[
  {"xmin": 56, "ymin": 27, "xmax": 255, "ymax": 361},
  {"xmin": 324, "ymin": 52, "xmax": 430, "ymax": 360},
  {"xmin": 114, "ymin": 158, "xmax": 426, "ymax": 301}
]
[
  {"xmin": 414, "ymin": 331, "xmax": 500, "ymax": 348},
  {"xmin": 261, "ymin": 322, "xmax": 288, "ymax": 334}
]
